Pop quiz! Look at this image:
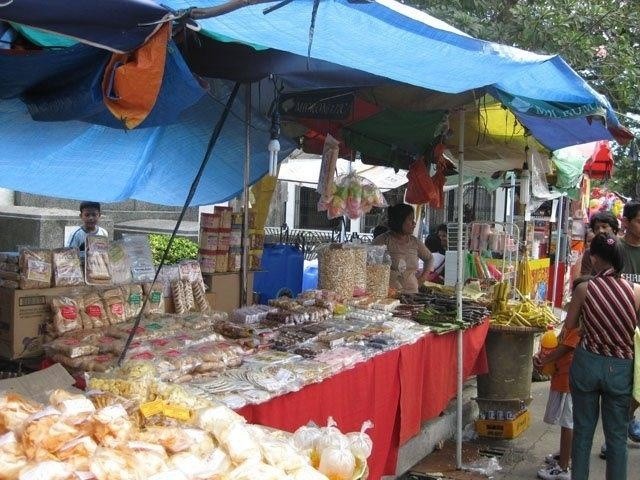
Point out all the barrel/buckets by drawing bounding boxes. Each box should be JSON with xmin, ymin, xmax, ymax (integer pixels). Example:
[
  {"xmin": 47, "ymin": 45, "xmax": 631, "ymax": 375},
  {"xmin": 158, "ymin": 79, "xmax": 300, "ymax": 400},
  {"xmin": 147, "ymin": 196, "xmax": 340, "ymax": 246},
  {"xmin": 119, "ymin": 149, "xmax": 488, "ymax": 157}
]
[{"xmin": 477, "ymin": 333, "xmax": 533, "ymax": 404}]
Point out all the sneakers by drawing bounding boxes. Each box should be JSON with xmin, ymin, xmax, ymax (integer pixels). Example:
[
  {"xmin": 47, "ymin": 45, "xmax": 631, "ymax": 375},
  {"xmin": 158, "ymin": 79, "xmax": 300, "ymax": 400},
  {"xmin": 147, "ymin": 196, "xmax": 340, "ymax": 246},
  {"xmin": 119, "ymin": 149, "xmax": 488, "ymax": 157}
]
[
  {"xmin": 545, "ymin": 451, "xmax": 572, "ymax": 469},
  {"xmin": 537, "ymin": 461, "xmax": 572, "ymax": 480},
  {"xmin": 600, "ymin": 443, "xmax": 607, "ymax": 456},
  {"xmin": 627, "ymin": 419, "xmax": 640, "ymax": 443}
]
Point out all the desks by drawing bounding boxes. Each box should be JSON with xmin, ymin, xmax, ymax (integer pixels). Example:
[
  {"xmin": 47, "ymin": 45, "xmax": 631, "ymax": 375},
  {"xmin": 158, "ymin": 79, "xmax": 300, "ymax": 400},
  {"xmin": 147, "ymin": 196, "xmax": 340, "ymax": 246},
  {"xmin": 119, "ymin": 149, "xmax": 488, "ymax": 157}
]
[{"xmin": 36, "ymin": 296, "xmax": 491, "ymax": 480}]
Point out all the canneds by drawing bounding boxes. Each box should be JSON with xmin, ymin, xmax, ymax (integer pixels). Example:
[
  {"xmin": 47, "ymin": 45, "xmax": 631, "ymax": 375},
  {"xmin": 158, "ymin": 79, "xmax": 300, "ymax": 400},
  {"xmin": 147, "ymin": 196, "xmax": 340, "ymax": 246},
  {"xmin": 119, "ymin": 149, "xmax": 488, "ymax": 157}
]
[
  {"xmin": 479, "ymin": 409, "xmax": 513, "ymax": 421},
  {"xmin": 520, "ymin": 400, "xmax": 525, "ymax": 410}
]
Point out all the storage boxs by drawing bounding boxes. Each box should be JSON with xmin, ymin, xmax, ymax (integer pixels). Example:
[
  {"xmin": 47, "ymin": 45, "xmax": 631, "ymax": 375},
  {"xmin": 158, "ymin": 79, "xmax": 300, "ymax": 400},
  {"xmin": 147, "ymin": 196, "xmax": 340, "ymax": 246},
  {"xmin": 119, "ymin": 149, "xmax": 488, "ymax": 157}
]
[
  {"xmin": 204, "ymin": 273, "xmax": 254, "ymax": 312},
  {"xmin": 164, "ymin": 293, "xmax": 216, "ymax": 314},
  {"xmin": 0, "ymin": 283, "xmax": 87, "ymax": 359}
]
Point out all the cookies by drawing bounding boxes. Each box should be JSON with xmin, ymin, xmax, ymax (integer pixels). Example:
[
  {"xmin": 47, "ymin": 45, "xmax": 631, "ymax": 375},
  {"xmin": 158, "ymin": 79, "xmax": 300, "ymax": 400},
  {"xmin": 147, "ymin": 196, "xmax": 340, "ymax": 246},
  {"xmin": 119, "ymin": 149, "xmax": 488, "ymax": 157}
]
[{"xmin": 191, "ymin": 369, "xmax": 281, "ymax": 410}]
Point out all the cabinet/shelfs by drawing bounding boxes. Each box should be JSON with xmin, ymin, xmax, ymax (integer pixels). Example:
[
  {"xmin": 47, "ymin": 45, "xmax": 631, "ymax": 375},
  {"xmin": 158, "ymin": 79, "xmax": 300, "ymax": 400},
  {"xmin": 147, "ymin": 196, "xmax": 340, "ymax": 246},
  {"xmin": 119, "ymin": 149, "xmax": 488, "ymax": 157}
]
[{"xmin": 465, "ymin": 220, "xmax": 519, "ymax": 299}]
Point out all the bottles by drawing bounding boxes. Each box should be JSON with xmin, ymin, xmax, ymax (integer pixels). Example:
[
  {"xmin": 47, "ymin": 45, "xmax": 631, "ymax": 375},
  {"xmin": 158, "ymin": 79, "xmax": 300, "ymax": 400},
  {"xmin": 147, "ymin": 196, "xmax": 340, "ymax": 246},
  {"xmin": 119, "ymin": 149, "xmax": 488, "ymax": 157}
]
[{"xmin": 539, "ymin": 324, "xmax": 559, "ymax": 376}]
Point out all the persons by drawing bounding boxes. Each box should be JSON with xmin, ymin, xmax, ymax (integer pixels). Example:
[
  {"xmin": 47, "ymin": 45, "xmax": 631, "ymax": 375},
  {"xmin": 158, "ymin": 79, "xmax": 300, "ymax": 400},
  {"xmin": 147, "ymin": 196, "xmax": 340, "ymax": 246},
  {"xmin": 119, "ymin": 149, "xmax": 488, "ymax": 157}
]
[
  {"xmin": 63, "ymin": 200, "xmax": 109, "ymax": 268},
  {"xmin": 537, "ymin": 202, "xmax": 640, "ymax": 480},
  {"xmin": 374, "ymin": 226, "xmax": 386, "ymax": 238},
  {"xmin": 371, "ymin": 204, "xmax": 433, "ymax": 298},
  {"xmin": 420, "ymin": 208, "xmax": 429, "ymax": 234},
  {"xmin": 436, "ymin": 223, "xmax": 447, "ymax": 256},
  {"xmin": 420, "ymin": 236, "xmax": 446, "ymax": 285}
]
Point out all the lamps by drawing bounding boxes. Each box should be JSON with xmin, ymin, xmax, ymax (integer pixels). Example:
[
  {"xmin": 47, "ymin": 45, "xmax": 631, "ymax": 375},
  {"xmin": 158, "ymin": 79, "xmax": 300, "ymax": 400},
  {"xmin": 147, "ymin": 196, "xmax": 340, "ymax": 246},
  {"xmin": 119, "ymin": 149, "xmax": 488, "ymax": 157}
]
[
  {"xmin": 520, "ymin": 132, "xmax": 530, "ymax": 205},
  {"xmin": 266, "ymin": 71, "xmax": 284, "ymax": 177}
]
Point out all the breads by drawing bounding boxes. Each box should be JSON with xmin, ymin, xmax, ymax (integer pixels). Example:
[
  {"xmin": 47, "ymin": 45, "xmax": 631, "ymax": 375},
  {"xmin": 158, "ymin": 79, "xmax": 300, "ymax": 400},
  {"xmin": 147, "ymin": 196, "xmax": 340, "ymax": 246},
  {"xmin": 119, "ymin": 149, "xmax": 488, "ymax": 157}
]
[{"xmin": 0, "ymin": 390, "xmax": 373, "ymax": 480}]
